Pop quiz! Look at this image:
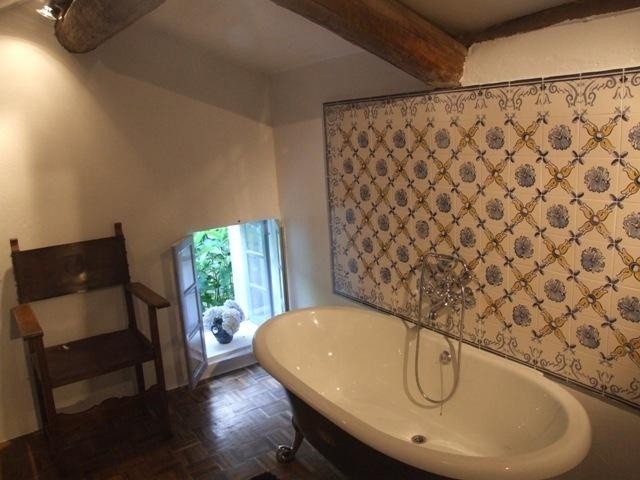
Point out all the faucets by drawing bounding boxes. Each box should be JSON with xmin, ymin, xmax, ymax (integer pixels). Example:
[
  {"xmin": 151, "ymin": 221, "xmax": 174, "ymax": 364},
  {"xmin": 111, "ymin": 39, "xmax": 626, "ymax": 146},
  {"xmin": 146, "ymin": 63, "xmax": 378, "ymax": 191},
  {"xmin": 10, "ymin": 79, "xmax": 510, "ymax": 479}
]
[{"xmin": 423, "ymin": 272, "xmax": 460, "ymax": 320}]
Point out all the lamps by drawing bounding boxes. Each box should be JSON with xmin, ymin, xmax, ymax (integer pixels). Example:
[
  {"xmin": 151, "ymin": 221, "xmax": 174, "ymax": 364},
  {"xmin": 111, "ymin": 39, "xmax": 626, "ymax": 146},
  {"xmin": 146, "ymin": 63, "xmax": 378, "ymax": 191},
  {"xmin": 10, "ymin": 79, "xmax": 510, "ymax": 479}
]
[{"xmin": 35, "ymin": 3, "xmax": 60, "ymax": 25}]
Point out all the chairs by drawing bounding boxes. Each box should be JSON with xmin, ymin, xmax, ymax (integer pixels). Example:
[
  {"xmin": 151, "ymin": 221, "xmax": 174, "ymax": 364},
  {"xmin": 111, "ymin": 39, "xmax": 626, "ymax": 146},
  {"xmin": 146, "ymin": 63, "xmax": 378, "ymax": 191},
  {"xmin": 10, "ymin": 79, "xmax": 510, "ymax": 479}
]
[{"xmin": 8, "ymin": 223, "xmax": 171, "ymax": 455}]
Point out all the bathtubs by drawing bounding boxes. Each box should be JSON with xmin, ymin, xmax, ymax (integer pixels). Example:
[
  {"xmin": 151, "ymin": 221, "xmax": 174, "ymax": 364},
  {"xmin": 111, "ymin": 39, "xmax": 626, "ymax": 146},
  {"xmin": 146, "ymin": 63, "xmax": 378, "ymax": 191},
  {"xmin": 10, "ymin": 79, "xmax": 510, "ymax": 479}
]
[{"xmin": 252, "ymin": 305, "xmax": 593, "ymax": 480}]
